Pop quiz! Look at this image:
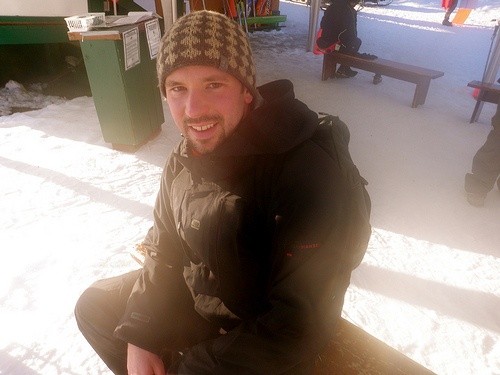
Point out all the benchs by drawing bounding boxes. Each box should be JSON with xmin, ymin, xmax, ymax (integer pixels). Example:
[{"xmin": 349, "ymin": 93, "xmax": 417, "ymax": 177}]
[
  {"xmin": 129, "ymin": 239, "xmax": 435, "ymax": 375},
  {"xmin": 467, "ymin": 79, "xmax": 500, "ymax": 123},
  {"xmin": 321, "ymin": 50, "xmax": 444, "ymax": 107}
]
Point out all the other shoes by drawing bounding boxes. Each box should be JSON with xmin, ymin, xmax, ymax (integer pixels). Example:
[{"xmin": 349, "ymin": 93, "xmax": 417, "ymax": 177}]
[
  {"xmin": 465, "ymin": 173, "xmax": 488, "ymax": 207},
  {"xmin": 337, "ymin": 65, "xmax": 358, "ymax": 78},
  {"xmin": 443, "ymin": 21, "xmax": 453, "ymax": 26}
]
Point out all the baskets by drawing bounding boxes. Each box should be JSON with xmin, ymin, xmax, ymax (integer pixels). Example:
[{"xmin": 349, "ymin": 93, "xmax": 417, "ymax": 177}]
[{"xmin": 64, "ymin": 13, "xmax": 106, "ymax": 32}]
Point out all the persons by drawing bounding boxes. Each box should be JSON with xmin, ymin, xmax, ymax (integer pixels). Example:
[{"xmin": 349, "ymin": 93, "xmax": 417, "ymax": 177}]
[
  {"xmin": 316, "ymin": 0, "xmax": 362, "ymax": 76},
  {"xmin": 442, "ymin": 0, "xmax": 458, "ymax": 26},
  {"xmin": 74, "ymin": 8, "xmax": 372, "ymax": 374},
  {"xmin": 466, "ymin": 95, "xmax": 500, "ymax": 207}
]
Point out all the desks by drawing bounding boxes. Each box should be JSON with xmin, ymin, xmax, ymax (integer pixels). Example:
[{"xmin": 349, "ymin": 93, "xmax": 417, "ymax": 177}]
[{"xmin": 67, "ymin": 16, "xmax": 165, "ymax": 154}]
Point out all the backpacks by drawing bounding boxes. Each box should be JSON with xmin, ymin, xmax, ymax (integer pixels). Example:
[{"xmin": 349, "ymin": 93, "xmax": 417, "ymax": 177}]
[{"xmin": 318, "ymin": 110, "xmax": 373, "ymax": 273}]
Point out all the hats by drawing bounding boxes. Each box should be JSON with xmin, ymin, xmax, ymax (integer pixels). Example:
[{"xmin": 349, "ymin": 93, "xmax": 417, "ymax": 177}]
[{"xmin": 155, "ymin": 10, "xmax": 258, "ymax": 113}]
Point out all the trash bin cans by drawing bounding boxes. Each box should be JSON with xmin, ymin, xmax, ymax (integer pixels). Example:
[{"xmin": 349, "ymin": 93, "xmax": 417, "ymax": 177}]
[{"xmin": 67, "ymin": 12, "xmax": 165, "ymax": 154}]
